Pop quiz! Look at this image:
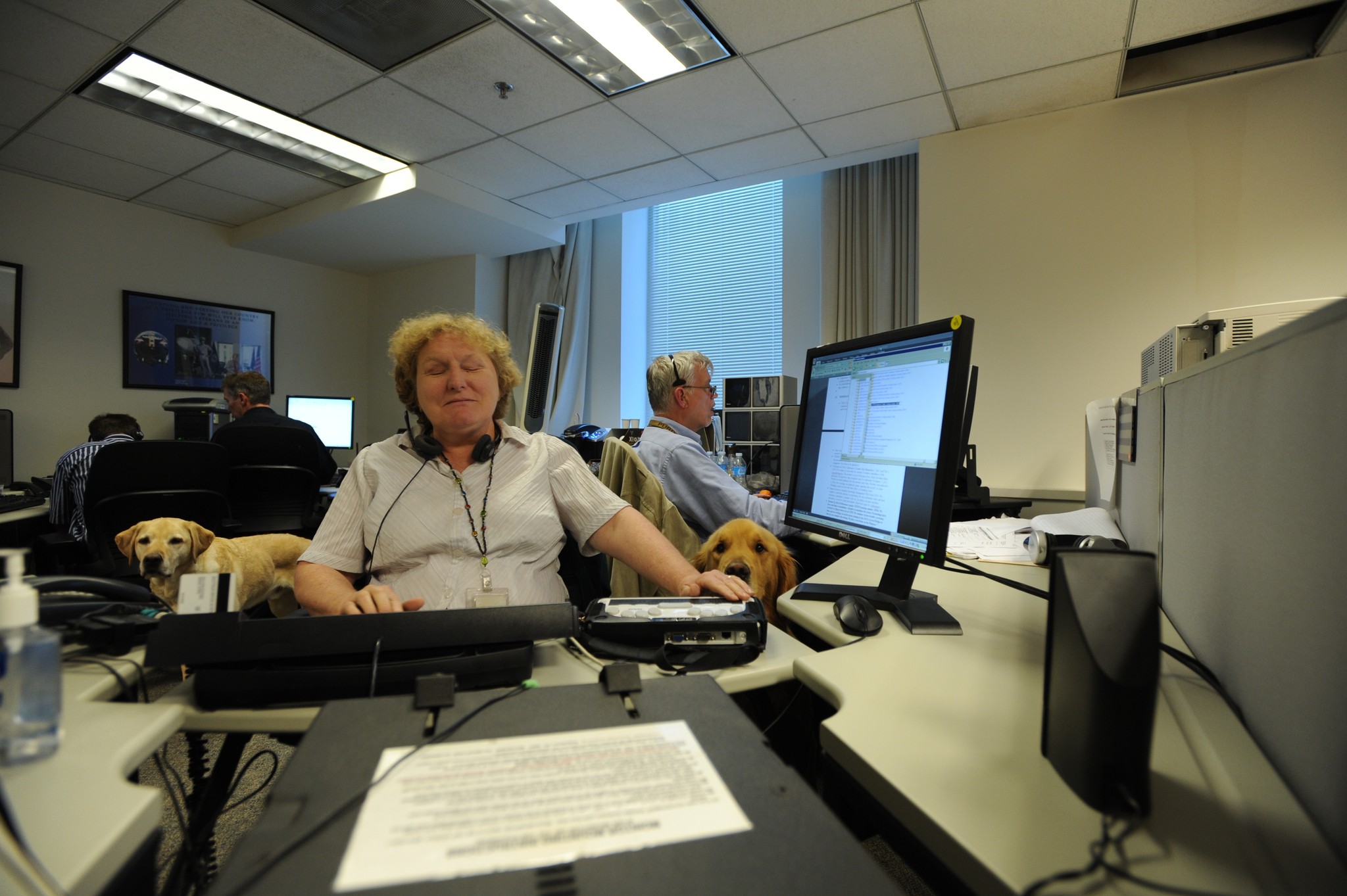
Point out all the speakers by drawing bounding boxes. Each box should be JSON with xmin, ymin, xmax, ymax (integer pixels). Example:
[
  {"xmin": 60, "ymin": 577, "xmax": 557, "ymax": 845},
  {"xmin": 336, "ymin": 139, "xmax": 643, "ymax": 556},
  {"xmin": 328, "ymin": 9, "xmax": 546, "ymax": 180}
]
[{"xmin": 1039, "ymin": 546, "xmax": 1163, "ymax": 819}]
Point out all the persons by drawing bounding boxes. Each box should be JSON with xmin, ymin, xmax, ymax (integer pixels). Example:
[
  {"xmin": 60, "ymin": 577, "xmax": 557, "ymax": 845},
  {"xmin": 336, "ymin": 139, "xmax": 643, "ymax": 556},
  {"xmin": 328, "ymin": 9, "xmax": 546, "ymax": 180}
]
[
  {"xmin": 294, "ymin": 311, "xmax": 754, "ymax": 620},
  {"xmin": 631, "ymin": 351, "xmax": 806, "ymax": 538},
  {"xmin": 49, "ymin": 412, "xmax": 145, "ymax": 541},
  {"xmin": 211, "ymin": 373, "xmax": 338, "ymax": 486}
]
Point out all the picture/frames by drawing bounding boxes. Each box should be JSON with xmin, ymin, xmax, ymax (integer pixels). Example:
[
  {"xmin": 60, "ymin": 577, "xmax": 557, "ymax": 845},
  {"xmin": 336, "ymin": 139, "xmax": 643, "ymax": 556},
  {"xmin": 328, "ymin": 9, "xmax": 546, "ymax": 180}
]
[{"xmin": 0, "ymin": 260, "xmax": 24, "ymax": 388}]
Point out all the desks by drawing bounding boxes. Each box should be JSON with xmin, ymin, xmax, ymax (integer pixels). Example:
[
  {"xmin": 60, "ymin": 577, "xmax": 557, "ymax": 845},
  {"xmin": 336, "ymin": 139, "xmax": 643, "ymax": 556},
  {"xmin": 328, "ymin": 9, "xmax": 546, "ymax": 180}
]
[{"xmin": 1, "ymin": 499, "xmax": 1347, "ymax": 894}]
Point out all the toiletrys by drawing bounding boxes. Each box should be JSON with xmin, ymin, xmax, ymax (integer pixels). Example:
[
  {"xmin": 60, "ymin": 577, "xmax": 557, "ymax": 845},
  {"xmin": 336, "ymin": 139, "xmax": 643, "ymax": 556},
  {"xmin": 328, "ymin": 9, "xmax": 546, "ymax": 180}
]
[{"xmin": 0, "ymin": 546, "xmax": 63, "ymax": 767}]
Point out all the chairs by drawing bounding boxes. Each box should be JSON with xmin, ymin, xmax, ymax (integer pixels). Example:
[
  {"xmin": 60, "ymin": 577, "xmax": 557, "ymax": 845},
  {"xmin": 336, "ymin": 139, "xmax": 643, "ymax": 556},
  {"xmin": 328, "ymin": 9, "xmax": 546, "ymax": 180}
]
[{"xmin": 79, "ymin": 425, "xmax": 321, "ymax": 591}]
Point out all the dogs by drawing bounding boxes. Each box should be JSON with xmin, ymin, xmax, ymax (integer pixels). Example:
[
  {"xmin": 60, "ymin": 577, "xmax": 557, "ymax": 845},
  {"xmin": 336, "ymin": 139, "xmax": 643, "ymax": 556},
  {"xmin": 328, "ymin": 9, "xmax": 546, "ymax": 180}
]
[
  {"xmin": 689, "ymin": 518, "xmax": 801, "ymax": 625},
  {"xmin": 116, "ymin": 516, "xmax": 312, "ymax": 618}
]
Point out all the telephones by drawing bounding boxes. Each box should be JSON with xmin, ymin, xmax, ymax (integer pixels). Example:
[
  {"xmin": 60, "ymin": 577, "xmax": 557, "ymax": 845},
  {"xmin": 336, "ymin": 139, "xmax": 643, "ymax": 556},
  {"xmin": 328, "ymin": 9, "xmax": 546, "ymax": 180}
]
[
  {"xmin": 0, "ymin": 574, "xmax": 161, "ymax": 623},
  {"xmin": 563, "ymin": 423, "xmax": 600, "ymax": 436},
  {"xmin": 9, "ymin": 480, "xmax": 45, "ymax": 497}
]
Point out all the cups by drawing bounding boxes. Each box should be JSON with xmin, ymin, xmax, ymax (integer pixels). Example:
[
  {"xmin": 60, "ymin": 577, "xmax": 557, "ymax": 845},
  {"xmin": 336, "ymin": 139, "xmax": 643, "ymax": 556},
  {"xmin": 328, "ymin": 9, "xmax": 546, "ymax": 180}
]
[
  {"xmin": 622, "ymin": 419, "xmax": 630, "ymax": 429},
  {"xmin": 631, "ymin": 419, "xmax": 640, "ymax": 428}
]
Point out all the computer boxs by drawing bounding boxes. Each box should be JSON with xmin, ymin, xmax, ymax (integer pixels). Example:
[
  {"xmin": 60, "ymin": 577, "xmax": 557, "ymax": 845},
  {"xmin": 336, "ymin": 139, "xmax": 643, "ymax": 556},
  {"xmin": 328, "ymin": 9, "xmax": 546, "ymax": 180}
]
[
  {"xmin": 174, "ymin": 412, "xmax": 230, "ymax": 442},
  {"xmin": 1, "ymin": 408, "xmax": 15, "ymax": 489},
  {"xmin": 206, "ymin": 662, "xmax": 905, "ymax": 896},
  {"xmin": 778, "ymin": 404, "xmax": 801, "ymax": 493}
]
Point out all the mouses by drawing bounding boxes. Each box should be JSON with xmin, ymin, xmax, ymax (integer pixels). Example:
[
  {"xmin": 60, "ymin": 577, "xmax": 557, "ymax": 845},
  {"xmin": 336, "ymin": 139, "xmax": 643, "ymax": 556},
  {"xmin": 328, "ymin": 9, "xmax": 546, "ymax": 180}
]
[{"xmin": 832, "ymin": 594, "xmax": 883, "ymax": 638}]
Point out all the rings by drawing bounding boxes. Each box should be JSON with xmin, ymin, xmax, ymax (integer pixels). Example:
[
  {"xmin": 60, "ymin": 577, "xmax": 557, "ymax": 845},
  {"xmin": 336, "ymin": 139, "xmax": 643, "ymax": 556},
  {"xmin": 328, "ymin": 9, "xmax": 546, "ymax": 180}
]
[{"xmin": 726, "ymin": 574, "xmax": 731, "ymax": 579}]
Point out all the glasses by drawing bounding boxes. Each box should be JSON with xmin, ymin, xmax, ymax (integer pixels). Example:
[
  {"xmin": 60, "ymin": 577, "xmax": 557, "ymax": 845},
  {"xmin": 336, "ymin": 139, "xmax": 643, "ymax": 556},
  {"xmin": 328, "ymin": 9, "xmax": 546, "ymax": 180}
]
[{"xmin": 681, "ymin": 384, "xmax": 718, "ymax": 400}]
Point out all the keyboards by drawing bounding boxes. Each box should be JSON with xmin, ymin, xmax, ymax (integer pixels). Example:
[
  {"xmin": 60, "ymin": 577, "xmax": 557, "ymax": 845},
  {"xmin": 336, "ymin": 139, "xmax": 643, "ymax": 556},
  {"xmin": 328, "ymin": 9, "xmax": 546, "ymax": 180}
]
[{"xmin": 770, "ymin": 491, "xmax": 788, "ymax": 502}]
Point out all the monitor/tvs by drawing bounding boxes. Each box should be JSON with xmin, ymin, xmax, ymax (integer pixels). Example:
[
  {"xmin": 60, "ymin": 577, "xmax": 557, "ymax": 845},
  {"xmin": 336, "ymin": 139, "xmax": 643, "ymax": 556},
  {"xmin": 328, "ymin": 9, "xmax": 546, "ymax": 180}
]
[
  {"xmin": 285, "ymin": 394, "xmax": 355, "ymax": 450},
  {"xmin": 144, "ymin": 601, "xmax": 579, "ymax": 714},
  {"xmin": 783, "ymin": 313, "xmax": 981, "ymax": 635}
]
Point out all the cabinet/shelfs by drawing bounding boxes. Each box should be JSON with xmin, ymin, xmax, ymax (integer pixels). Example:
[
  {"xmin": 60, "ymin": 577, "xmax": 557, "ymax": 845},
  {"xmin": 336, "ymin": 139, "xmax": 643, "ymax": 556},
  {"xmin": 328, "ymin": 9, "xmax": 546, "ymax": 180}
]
[{"xmin": 722, "ymin": 375, "xmax": 798, "ymax": 479}]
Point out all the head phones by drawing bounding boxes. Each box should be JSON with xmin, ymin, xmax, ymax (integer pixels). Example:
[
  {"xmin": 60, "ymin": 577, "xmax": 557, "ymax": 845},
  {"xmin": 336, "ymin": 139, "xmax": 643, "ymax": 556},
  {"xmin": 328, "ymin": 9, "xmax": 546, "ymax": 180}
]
[
  {"xmin": 404, "ymin": 408, "xmax": 503, "ymax": 465},
  {"xmin": 87, "ymin": 430, "xmax": 144, "ymax": 443}
]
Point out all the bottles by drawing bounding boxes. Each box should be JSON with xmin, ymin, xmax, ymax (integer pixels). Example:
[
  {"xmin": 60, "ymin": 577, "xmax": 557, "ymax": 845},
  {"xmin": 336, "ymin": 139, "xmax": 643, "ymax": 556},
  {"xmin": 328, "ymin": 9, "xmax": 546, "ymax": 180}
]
[
  {"xmin": 730, "ymin": 453, "xmax": 746, "ymax": 490},
  {"xmin": 707, "ymin": 451, "xmax": 716, "ymax": 463},
  {"xmin": 716, "ymin": 451, "xmax": 729, "ymax": 475}
]
